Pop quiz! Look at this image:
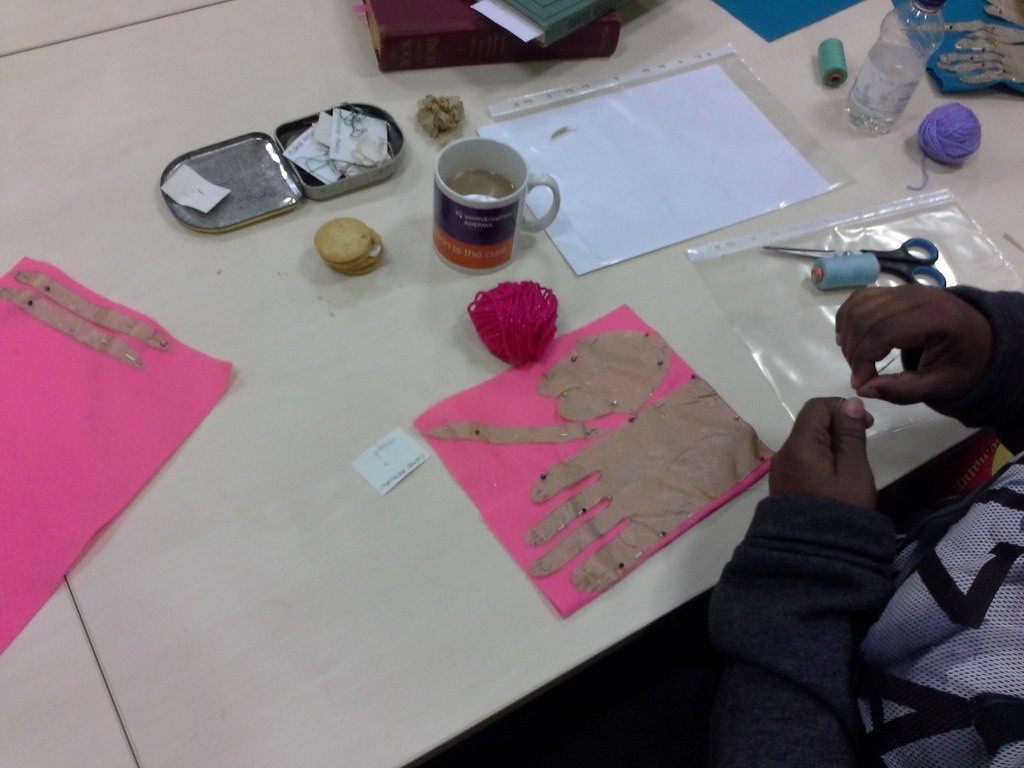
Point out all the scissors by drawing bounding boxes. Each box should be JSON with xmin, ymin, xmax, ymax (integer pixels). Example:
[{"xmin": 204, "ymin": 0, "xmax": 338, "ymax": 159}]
[{"xmin": 764, "ymin": 236, "xmax": 948, "ymax": 290}]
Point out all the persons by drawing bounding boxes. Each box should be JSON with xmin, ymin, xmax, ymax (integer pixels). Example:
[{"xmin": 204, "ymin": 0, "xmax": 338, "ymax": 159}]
[{"xmin": 699, "ymin": 283, "xmax": 1024, "ymax": 767}]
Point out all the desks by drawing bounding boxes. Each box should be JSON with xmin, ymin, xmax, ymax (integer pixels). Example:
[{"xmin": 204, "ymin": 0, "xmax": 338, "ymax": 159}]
[{"xmin": 0, "ymin": 0, "xmax": 1024, "ymax": 768}]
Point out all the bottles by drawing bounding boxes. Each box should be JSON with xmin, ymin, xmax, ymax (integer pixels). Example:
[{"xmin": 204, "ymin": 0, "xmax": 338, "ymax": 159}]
[{"xmin": 844, "ymin": 0, "xmax": 946, "ymax": 135}]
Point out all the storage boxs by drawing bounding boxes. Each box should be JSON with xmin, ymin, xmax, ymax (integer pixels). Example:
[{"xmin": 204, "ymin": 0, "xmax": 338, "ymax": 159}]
[{"xmin": 161, "ymin": 105, "xmax": 406, "ymax": 234}]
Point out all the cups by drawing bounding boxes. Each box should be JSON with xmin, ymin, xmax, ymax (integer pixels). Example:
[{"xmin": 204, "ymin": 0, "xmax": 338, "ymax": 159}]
[{"xmin": 432, "ymin": 137, "xmax": 562, "ymax": 275}]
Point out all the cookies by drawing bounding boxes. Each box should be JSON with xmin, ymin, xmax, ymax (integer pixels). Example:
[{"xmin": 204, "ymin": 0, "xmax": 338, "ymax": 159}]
[
  {"xmin": 315, "ymin": 217, "xmax": 371, "ymax": 266},
  {"xmin": 326, "ymin": 227, "xmax": 381, "ymax": 275}
]
